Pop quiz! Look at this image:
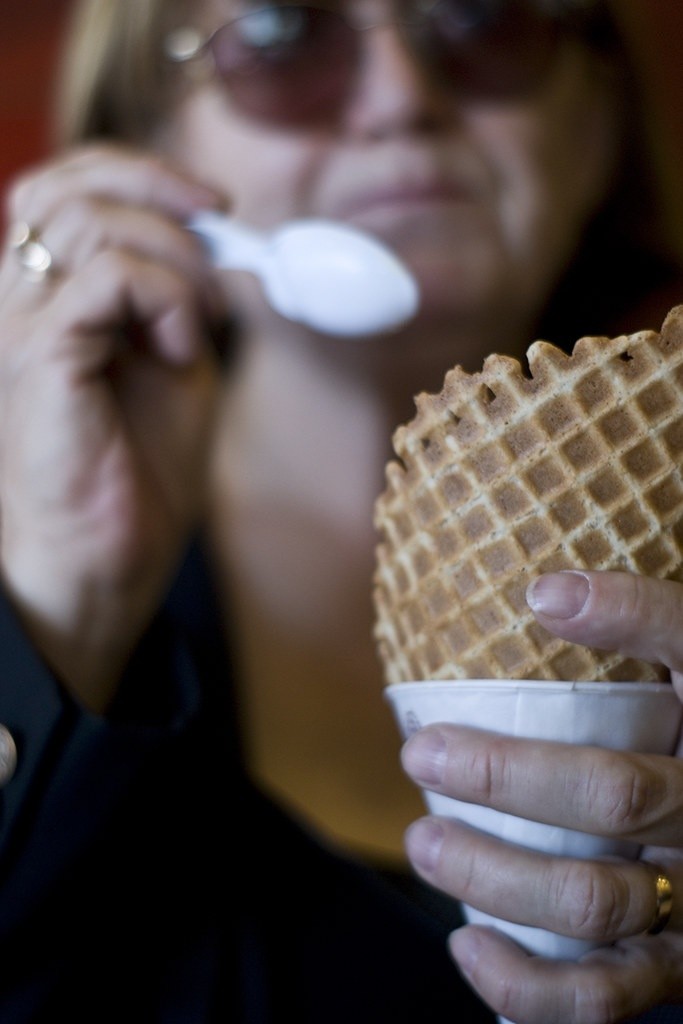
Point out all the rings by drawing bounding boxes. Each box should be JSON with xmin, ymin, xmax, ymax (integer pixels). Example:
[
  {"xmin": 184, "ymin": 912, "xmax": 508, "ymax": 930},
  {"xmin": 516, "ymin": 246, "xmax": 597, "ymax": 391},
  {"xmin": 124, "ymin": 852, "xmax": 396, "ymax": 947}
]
[
  {"xmin": 19, "ymin": 232, "xmax": 54, "ymax": 275},
  {"xmin": 642, "ymin": 862, "xmax": 674, "ymax": 936}
]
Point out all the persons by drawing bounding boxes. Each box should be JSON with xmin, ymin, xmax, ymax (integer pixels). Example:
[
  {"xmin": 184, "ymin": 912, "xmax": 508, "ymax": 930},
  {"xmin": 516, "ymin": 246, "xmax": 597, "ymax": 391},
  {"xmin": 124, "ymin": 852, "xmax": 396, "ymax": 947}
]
[{"xmin": 0, "ymin": 0, "xmax": 683, "ymax": 1024}]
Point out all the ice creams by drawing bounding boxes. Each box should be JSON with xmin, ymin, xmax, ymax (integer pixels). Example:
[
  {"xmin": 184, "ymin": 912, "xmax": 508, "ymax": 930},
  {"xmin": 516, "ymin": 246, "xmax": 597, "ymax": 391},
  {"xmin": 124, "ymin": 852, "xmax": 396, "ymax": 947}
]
[{"xmin": 368, "ymin": 304, "xmax": 683, "ymax": 1022}]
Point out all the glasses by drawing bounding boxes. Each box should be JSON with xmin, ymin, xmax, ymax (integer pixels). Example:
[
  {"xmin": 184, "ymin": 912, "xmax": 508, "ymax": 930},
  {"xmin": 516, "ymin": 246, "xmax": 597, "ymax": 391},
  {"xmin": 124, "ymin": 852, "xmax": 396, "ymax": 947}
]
[{"xmin": 169, "ymin": 0, "xmax": 582, "ymax": 122}]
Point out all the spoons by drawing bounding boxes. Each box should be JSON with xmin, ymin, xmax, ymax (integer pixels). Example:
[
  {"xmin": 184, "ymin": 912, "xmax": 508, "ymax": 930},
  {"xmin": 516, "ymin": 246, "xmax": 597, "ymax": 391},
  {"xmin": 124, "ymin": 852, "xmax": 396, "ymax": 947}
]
[{"xmin": 191, "ymin": 210, "xmax": 417, "ymax": 339}]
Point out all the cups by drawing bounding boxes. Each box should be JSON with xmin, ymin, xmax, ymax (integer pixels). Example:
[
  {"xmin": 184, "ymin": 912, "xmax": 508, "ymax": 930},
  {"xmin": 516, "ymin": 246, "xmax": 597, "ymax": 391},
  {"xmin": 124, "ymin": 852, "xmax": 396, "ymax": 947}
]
[{"xmin": 386, "ymin": 682, "xmax": 681, "ymax": 1024}]
